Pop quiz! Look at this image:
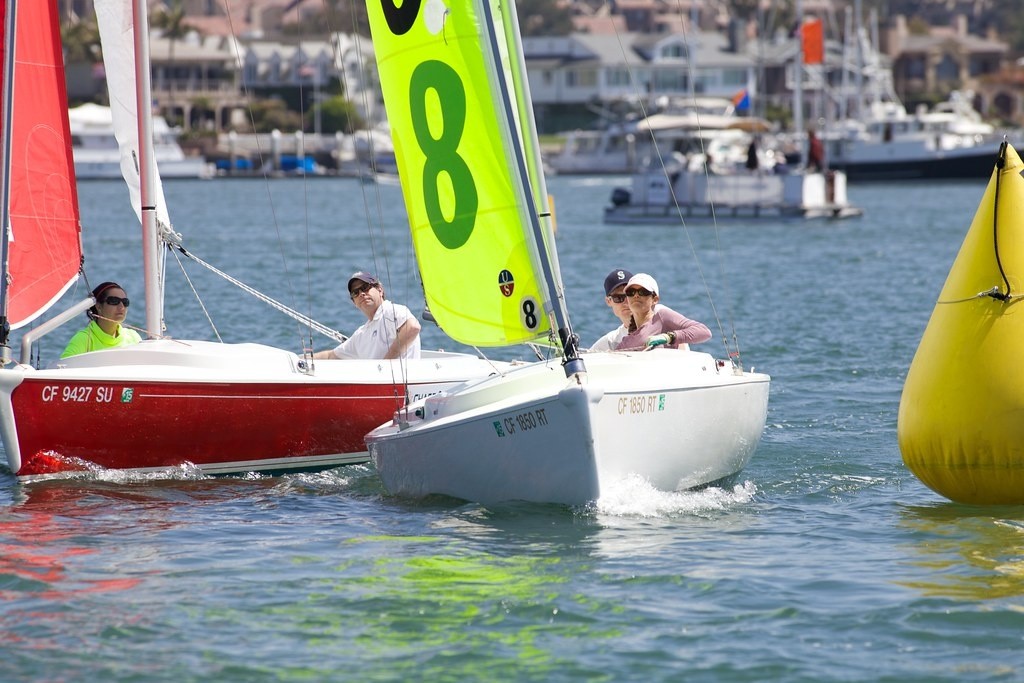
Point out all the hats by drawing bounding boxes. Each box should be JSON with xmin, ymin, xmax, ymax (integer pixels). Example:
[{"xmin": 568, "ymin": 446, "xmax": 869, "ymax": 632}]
[
  {"xmin": 347, "ymin": 271, "xmax": 378, "ymax": 292},
  {"xmin": 604, "ymin": 268, "xmax": 634, "ymax": 297},
  {"xmin": 92, "ymin": 281, "xmax": 119, "ymax": 298},
  {"xmin": 623, "ymin": 272, "xmax": 659, "ymax": 297}
]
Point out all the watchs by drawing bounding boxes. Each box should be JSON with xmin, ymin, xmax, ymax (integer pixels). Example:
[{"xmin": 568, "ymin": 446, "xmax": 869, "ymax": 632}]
[{"xmin": 666, "ymin": 331, "xmax": 676, "ymax": 344}]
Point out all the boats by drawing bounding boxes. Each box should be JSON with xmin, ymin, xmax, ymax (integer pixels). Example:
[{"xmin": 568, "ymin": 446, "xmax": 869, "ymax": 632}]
[
  {"xmin": 779, "ymin": 112, "xmax": 1024, "ymax": 180},
  {"xmin": 548, "ymin": 95, "xmax": 739, "ymax": 177},
  {"xmin": 329, "ymin": 127, "xmax": 398, "ymax": 175},
  {"xmin": 64, "ymin": 104, "xmax": 217, "ymax": 180},
  {"xmin": 603, "ymin": 115, "xmax": 864, "ymax": 223},
  {"xmin": 356, "ymin": 170, "xmax": 401, "ymax": 188}
]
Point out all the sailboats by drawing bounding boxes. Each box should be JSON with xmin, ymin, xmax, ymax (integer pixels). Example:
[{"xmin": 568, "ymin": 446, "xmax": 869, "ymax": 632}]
[
  {"xmin": 0, "ymin": 1, "xmax": 535, "ymax": 483},
  {"xmin": 354, "ymin": 1, "xmax": 770, "ymax": 515}
]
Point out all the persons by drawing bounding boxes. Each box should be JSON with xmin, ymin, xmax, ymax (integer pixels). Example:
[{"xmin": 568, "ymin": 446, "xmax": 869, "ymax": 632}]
[
  {"xmin": 589, "ymin": 269, "xmax": 691, "ymax": 351},
  {"xmin": 806, "ymin": 131, "xmax": 824, "ymax": 172},
  {"xmin": 607, "ymin": 273, "xmax": 713, "ymax": 350},
  {"xmin": 60, "ymin": 282, "xmax": 143, "ymax": 359},
  {"xmin": 746, "ymin": 136, "xmax": 759, "ymax": 175},
  {"xmin": 297, "ymin": 271, "xmax": 422, "ymax": 360}
]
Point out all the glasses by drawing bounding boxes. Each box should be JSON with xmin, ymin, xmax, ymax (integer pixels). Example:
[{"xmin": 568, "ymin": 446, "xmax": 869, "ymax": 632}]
[
  {"xmin": 607, "ymin": 293, "xmax": 626, "ymax": 303},
  {"xmin": 101, "ymin": 296, "xmax": 129, "ymax": 307},
  {"xmin": 625, "ymin": 286, "xmax": 651, "ymax": 297},
  {"xmin": 350, "ymin": 282, "xmax": 371, "ymax": 298}
]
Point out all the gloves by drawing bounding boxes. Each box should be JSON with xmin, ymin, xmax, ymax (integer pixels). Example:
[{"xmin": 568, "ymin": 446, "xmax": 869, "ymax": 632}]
[{"xmin": 644, "ymin": 333, "xmax": 672, "ymax": 348}]
